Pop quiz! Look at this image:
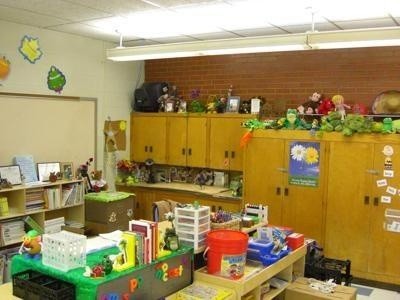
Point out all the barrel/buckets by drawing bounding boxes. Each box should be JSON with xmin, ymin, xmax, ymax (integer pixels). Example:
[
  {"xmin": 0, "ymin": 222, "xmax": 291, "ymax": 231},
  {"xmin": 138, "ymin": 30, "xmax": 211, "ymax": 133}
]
[{"xmin": 203, "ymin": 231, "xmax": 249, "ymax": 280}]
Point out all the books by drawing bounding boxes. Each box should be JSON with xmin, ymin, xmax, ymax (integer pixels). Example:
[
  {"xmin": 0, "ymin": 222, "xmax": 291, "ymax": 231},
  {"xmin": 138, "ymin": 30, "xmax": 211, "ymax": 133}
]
[
  {"xmin": 123, "ymin": 219, "xmax": 161, "ymax": 266},
  {"xmin": 1, "ymin": 183, "xmax": 86, "ymax": 286}
]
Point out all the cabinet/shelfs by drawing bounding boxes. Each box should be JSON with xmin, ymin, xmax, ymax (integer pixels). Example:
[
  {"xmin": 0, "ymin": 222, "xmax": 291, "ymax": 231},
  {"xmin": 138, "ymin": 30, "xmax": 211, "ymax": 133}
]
[{"xmin": 0, "ymin": 111, "xmax": 400, "ymax": 300}]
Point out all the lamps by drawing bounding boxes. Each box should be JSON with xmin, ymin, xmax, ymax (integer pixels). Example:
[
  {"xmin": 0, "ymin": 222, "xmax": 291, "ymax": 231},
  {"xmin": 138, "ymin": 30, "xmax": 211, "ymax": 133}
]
[{"xmin": 106, "ymin": 6, "xmax": 400, "ymax": 62}]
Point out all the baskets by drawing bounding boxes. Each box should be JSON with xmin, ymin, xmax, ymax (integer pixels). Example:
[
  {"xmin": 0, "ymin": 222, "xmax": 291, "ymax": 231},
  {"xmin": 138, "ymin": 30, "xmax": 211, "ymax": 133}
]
[{"xmin": 12, "ymin": 270, "xmax": 76, "ymax": 299}]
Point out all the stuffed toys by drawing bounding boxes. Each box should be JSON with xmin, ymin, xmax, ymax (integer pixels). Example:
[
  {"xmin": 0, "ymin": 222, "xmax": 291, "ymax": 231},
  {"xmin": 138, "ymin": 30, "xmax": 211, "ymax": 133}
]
[
  {"xmin": 297, "ymin": 91, "xmax": 322, "ymax": 114},
  {"xmin": 314, "ymin": 97, "xmax": 334, "ymax": 114},
  {"xmin": 332, "ymin": 94, "xmax": 350, "ymax": 123}
]
